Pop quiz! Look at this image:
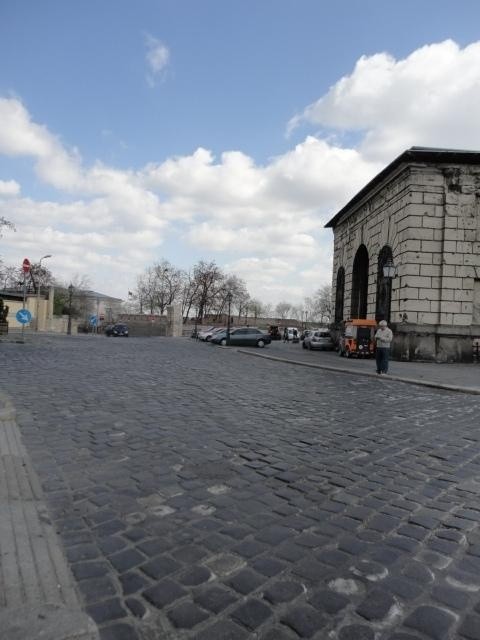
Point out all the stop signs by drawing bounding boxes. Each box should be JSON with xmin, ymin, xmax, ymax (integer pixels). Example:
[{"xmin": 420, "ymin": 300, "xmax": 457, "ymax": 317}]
[{"xmin": 23, "ymin": 258, "xmax": 30, "ymax": 273}]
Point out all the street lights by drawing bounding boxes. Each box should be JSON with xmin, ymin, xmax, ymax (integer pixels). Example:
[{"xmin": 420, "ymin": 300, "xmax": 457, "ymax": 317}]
[
  {"xmin": 226, "ymin": 293, "xmax": 233, "ymax": 346},
  {"xmin": 36, "ymin": 254, "xmax": 52, "ymax": 333},
  {"xmin": 67, "ymin": 284, "xmax": 76, "ymax": 334}
]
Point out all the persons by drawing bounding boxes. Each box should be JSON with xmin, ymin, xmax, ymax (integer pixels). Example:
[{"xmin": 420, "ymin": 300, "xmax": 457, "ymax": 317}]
[
  {"xmin": 373, "ymin": 319, "xmax": 394, "ymax": 374},
  {"xmin": 283, "ymin": 328, "xmax": 290, "ymax": 344}
]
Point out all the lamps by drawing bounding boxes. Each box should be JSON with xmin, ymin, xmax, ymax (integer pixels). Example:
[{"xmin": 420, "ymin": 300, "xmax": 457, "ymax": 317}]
[{"xmin": 383, "ymin": 258, "xmax": 401, "ymax": 279}]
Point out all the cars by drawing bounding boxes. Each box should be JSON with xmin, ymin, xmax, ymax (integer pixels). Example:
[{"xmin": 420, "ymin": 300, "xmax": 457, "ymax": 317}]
[
  {"xmin": 197, "ymin": 326, "xmax": 272, "ymax": 348},
  {"xmin": 285, "ymin": 319, "xmax": 377, "ymax": 358},
  {"xmin": 106, "ymin": 323, "xmax": 129, "ymax": 337}
]
